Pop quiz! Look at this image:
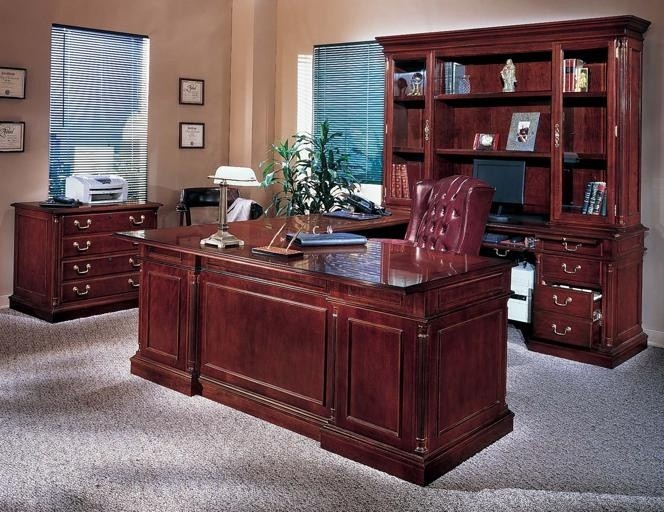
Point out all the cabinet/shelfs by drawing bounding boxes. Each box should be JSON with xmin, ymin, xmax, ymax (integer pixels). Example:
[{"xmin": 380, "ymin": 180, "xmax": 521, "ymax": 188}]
[
  {"xmin": 8, "ymin": 202, "xmax": 161, "ymax": 325},
  {"xmin": 374, "ymin": 12, "xmax": 652, "ymax": 370}
]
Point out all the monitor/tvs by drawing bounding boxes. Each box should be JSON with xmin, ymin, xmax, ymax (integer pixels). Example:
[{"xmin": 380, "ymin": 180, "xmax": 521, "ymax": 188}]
[{"xmin": 472, "ymin": 159, "xmax": 526, "ymax": 222}]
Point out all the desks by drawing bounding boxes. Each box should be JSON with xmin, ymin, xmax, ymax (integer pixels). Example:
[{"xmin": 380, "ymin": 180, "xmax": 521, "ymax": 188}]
[{"xmin": 114, "ymin": 208, "xmax": 515, "ymax": 487}]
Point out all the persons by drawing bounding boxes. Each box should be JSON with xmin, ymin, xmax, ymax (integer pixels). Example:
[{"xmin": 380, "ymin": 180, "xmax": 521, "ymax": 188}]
[{"xmin": 499, "ymin": 58, "xmax": 518, "ymax": 93}]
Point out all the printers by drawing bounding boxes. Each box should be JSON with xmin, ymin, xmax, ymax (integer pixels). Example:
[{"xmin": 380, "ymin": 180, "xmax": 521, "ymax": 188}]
[{"xmin": 65, "ymin": 172, "xmax": 128, "ymax": 205}]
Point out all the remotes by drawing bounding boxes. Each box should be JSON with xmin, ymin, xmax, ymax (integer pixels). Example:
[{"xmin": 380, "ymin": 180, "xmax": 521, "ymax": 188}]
[{"xmin": 53, "ymin": 195, "xmax": 75, "ymax": 203}]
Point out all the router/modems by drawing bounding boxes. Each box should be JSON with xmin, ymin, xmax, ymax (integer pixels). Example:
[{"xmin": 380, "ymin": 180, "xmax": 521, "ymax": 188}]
[{"xmin": 254, "ymin": 223, "xmax": 306, "ymax": 259}]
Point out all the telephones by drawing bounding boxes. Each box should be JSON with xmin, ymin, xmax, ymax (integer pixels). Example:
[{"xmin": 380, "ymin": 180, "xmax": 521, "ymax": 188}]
[{"xmin": 344, "ymin": 193, "xmax": 385, "ymax": 214}]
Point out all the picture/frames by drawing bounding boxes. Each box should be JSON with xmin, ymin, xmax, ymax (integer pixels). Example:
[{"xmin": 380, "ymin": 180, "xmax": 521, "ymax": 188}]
[
  {"xmin": 176, "ymin": 76, "xmax": 206, "ymax": 150},
  {"xmin": 0, "ymin": 65, "xmax": 27, "ymax": 154},
  {"xmin": 505, "ymin": 112, "xmax": 542, "ymax": 153}
]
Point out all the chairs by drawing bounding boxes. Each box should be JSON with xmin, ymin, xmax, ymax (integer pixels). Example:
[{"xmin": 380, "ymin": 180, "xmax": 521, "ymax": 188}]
[
  {"xmin": 401, "ymin": 174, "xmax": 496, "ymax": 257},
  {"xmin": 174, "ymin": 186, "xmax": 261, "ymax": 225}
]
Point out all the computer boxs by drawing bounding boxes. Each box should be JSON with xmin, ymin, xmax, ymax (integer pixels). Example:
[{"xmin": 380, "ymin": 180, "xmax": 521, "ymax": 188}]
[{"xmin": 507, "ymin": 265, "xmax": 535, "ymax": 323}]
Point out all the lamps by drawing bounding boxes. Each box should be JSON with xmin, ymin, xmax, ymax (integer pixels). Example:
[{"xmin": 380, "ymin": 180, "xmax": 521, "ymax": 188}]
[{"xmin": 198, "ymin": 165, "xmax": 262, "ymax": 249}]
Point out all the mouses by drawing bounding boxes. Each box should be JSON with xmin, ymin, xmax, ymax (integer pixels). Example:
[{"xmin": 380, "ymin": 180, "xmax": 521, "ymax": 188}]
[{"xmin": 511, "ymin": 235, "xmax": 524, "ymax": 243}]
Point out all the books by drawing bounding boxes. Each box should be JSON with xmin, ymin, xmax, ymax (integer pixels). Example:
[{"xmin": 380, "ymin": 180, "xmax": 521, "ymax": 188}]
[
  {"xmin": 286, "ymin": 230, "xmax": 367, "ymax": 247},
  {"xmin": 323, "ymin": 209, "xmax": 382, "ymax": 220},
  {"xmin": 592, "ymin": 182, "xmax": 606, "ymax": 216},
  {"xmin": 401, "ymin": 164, "xmax": 421, "ymax": 199},
  {"xmin": 586, "ymin": 182, "xmax": 599, "ymax": 214},
  {"xmin": 439, "ymin": 61, "xmax": 465, "ymax": 94},
  {"xmin": 562, "ymin": 59, "xmax": 607, "ymax": 92},
  {"xmin": 600, "ymin": 189, "xmax": 607, "ymax": 216},
  {"xmin": 581, "ymin": 181, "xmax": 594, "ymax": 215},
  {"xmin": 396, "ymin": 165, "xmax": 402, "ymax": 198},
  {"xmin": 391, "ymin": 163, "xmax": 396, "ymax": 197}
]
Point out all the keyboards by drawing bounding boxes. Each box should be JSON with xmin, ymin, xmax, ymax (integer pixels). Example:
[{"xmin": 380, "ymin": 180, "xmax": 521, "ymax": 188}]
[{"xmin": 482, "ymin": 232, "xmax": 509, "ymax": 243}]
